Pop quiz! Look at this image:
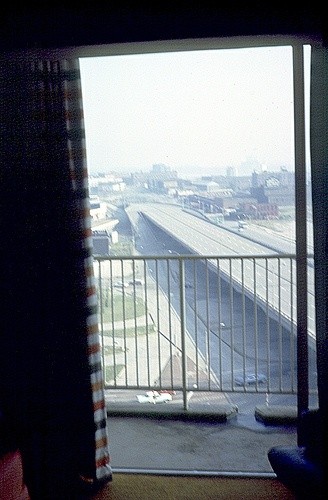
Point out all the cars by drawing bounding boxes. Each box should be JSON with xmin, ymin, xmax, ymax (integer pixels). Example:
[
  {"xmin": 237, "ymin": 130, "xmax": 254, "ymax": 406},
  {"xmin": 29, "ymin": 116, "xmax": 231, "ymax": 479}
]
[{"xmin": 236, "ymin": 373, "xmax": 267, "ymax": 385}]
